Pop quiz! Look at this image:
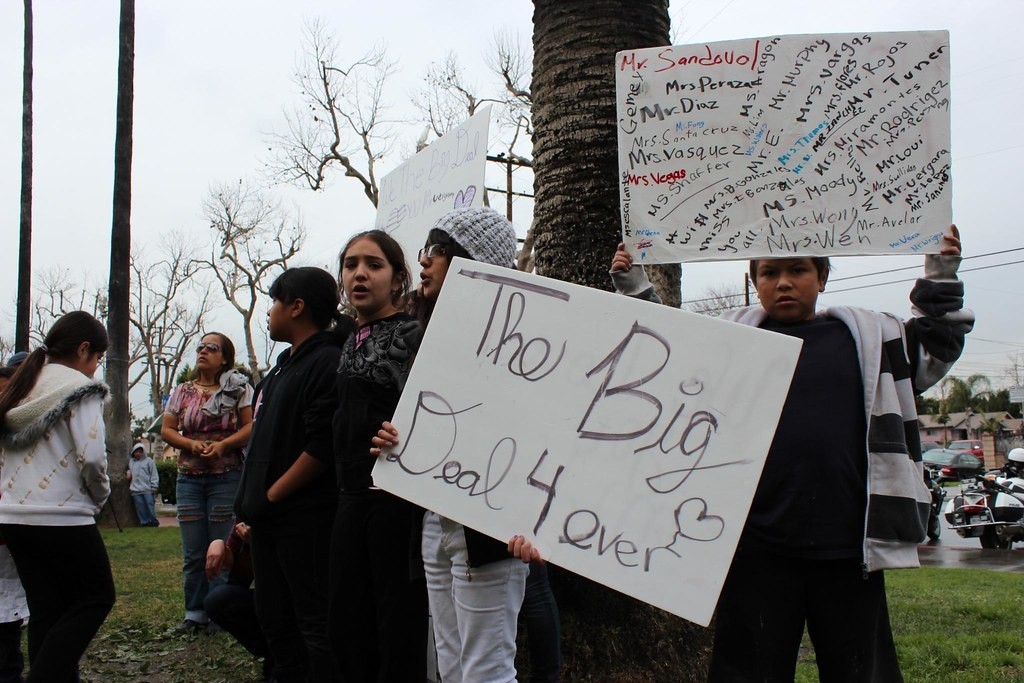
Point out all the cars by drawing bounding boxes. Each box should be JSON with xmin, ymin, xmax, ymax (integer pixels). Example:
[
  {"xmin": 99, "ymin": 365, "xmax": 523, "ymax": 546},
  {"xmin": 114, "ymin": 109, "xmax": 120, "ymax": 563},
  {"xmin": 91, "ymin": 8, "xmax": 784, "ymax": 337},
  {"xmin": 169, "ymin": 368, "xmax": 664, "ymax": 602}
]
[
  {"xmin": 921, "ymin": 448, "xmax": 984, "ymax": 486},
  {"xmin": 922, "ymin": 440, "xmax": 984, "ymax": 459}
]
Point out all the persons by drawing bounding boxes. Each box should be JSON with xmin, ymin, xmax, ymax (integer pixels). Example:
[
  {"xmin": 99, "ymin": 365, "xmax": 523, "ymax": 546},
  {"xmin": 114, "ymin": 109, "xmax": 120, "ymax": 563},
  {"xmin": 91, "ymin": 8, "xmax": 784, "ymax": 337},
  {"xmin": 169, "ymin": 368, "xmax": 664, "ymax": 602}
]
[
  {"xmin": 0, "ymin": 311, "xmax": 117, "ymax": 683},
  {"xmin": 609, "ymin": 225, "xmax": 975, "ymax": 683},
  {"xmin": 161, "ymin": 206, "xmax": 541, "ymax": 683},
  {"xmin": 126, "ymin": 443, "xmax": 159, "ymax": 527}
]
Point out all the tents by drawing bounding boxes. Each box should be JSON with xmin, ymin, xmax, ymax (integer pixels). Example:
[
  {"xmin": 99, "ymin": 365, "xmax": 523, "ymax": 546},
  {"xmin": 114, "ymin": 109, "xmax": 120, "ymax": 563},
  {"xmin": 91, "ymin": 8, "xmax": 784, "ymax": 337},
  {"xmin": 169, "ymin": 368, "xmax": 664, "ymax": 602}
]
[{"xmin": 147, "ymin": 412, "xmax": 175, "ymax": 453}]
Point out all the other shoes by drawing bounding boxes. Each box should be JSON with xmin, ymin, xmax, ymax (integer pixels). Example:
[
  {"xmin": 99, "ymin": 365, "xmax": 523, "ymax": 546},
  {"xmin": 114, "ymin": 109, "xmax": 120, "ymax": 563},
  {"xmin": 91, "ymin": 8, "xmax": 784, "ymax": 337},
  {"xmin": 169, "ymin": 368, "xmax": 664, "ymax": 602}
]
[
  {"xmin": 145, "ymin": 519, "xmax": 159, "ymax": 527},
  {"xmin": 175, "ymin": 619, "xmax": 209, "ymax": 636}
]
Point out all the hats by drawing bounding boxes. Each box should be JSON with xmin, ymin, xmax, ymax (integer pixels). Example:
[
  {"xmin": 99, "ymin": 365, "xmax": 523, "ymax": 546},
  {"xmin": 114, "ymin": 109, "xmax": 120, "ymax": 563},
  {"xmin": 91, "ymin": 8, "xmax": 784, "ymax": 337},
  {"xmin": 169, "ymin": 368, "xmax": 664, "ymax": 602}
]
[
  {"xmin": 429, "ymin": 206, "xmax": 516, "ymax": 268},
  {"xmin": 6, "ymin": 352, "xmax": 30, "ymax": 367}
]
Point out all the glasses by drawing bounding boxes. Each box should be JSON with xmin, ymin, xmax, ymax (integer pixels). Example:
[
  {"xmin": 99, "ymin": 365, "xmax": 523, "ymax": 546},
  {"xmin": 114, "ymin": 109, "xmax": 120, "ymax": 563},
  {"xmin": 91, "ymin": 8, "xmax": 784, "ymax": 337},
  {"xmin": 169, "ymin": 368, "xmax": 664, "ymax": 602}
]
[
  {"xmin": 418, "ymin": 244, "xmax": 454, "ymax": 261},
  {"xmin": 195, "ymin": 343, "xmax": 222, "ymax": 353}
]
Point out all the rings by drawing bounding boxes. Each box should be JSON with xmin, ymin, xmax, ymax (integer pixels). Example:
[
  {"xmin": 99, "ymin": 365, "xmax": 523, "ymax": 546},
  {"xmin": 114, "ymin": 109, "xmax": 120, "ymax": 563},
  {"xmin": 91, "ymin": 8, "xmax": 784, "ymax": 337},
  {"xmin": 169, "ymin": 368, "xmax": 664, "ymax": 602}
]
[
  {"xmin": 235, "ymin": 531, "xmax": 240, "ymax": 534},
  {"xmin": 193, "ymin": 452, "xmax": 196, "ymax": 455}
]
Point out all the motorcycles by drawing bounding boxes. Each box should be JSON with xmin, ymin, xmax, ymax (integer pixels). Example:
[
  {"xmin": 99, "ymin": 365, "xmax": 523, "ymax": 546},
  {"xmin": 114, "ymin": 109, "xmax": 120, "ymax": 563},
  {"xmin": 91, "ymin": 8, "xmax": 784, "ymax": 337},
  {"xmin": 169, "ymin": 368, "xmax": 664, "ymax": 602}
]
[
  {"xmin": 945, "ymin": 446, "xmax": 1023, "ymax": 549},
  {"xmin": 922, "ymin": 463, "xmax": 944, "ymax": 538}
]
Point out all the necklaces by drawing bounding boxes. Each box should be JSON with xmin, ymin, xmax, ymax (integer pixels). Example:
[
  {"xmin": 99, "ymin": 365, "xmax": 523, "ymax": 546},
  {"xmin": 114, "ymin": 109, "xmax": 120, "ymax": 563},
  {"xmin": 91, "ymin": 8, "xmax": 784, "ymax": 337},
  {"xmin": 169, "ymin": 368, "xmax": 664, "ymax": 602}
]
[{"xmin": 195, "ymin": 377, "xmax": 217, "ymax": 388}]
